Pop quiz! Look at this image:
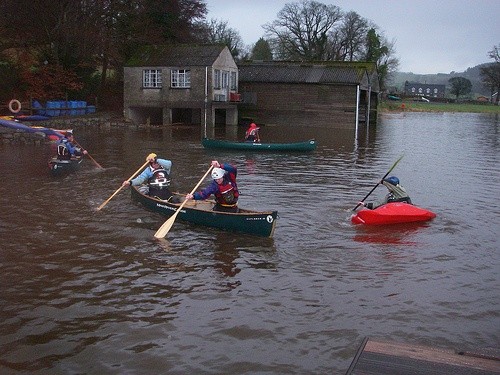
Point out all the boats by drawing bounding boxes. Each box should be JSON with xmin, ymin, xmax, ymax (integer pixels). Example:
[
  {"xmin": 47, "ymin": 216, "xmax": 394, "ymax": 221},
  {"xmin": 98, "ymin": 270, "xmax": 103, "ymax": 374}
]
[
  {"xmin": 49, "ymin": 149, "xmax": 83, "ymax": 175},
  {"xmin": 351, "ymin": 201, "xmax": 437, "ymax": 222},
  {"xmin": 130, "ymin": 178, "xmax": 278, "ymax": 239},
  {"xmin": 201, "ymin": 137, "xmax": 317, "ymax": 152}
]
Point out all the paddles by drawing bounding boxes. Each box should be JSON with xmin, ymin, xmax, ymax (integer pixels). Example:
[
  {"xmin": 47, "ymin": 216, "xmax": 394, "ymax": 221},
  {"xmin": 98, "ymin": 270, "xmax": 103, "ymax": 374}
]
[
  {"xmin": 352, "ymin": 153, "xmax": 405, "ymax": 211},
  {"xmin": 257, "ymin": 130, "xmax": 260, "ymax": 142},
  {"xmin": 73, "ymin": 138, "xmax": 102, "ymax": 169},
  {"xmin": 96, "ymin": 159, "xmax": 150, "ymax": 211},
  {"xmin": 153, "ymin": 164, "xmax": 215, "ymax": 239}
]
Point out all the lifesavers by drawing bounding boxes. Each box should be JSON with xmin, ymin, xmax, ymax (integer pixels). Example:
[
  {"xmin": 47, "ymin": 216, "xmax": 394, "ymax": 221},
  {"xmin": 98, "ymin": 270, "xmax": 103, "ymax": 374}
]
[{"xmin": 8, "ymin": 99, "xmax": 22, "ymax": 113}]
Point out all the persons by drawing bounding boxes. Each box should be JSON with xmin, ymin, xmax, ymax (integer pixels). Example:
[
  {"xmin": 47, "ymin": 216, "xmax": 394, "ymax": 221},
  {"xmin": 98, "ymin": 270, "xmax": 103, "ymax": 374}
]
[
  {"xmin": 56, "ymin": 131, "xmax": 88, "ymax": 162},
  {"xmin": 245, "ymin": 122, "xmax": 260, "ymax": 144},
  {"xmin": 358, "ymin": 176, "xmax": 412, "ymax": 211},
  {"xmin": 121, "ymin": 153, "xmax": 172, "ymax": 203},
  {"xmin": 186, "ymin": 160, "xmax": 240, "ymax": 213}
]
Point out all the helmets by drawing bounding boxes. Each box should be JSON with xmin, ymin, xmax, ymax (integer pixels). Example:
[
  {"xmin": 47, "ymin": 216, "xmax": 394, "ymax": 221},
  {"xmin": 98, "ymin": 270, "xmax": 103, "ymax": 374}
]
[
  {"xmin": 145, "ymin": 153, "xmax": 159, "ymax": 162},
  {"xmin": 211, "ymin": 167, "xmax": 225, "ymax": 180},
  {"xmin": 383, "ymin": 176, "xmax": 399, "ymax": 185}
]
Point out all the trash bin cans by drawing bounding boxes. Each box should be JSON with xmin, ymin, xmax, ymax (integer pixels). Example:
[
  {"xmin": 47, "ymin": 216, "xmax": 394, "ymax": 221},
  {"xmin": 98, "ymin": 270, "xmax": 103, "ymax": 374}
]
[{"xmin": 31, "ymin": 100, "xmax": 97, "ymax": 116}]
